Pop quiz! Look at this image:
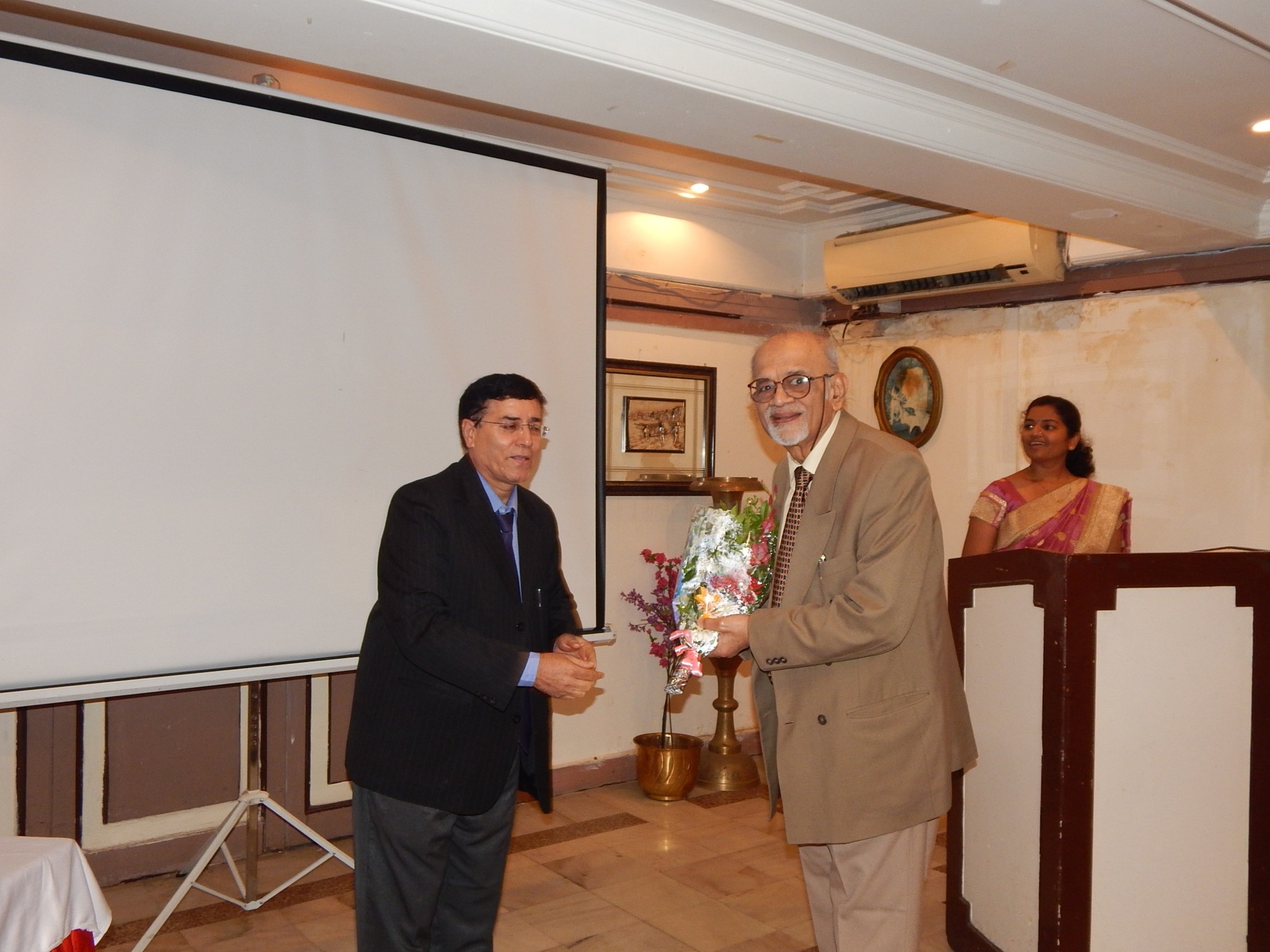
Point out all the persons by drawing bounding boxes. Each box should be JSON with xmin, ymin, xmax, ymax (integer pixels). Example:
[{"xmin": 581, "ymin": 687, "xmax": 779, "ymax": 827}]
[
  {"xmin": 696, "ymin": 323, "xmax": 978, "ymax": 952},
  {"xmin": 962, "ymin": 397, "xmax": 1133, "ymax": 558},
  {"xmin": 345, "ymin": 373, "xmax": 605, "ymax": 952}
]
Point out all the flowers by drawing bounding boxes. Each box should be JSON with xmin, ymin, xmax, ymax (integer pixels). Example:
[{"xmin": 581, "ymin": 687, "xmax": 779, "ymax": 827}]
[
  {"xmin": 642, "ymin": 504, "xmax": 783, "ymax": 696},
  {"xmin": 620, "ymin": 547, "xmax": 683, "ymax": 747}
]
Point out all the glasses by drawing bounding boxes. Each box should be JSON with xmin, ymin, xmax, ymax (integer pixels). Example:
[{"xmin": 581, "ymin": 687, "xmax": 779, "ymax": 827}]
[
  {"xmin": 471, "ymin": 419, "xmax": 550, "ymax": 438},
  {"xmin": 747, "ymin": 374, "xmax": 834, "ymax": 403}
]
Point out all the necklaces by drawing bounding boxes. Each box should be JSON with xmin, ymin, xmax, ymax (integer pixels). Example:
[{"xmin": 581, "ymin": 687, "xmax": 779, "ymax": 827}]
[{"xmin": 1030, "ymin": 466, "xmax": 1072, "ymax": 503}]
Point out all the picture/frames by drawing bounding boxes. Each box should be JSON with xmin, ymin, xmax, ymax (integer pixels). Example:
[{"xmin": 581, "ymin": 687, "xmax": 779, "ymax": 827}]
[
  {"xmin": 604, "ymin": 358, "xmax": 718, "ymax": 496},
  {"xmin": 875, "ymin": 346, "xmax": 942, "ymax": 449}
]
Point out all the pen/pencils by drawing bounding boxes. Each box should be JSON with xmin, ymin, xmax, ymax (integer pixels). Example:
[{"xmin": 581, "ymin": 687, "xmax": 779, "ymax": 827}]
[
  {"xmin": 538, "ymin": 589, "xmax": 541, "ymax": 607},
  {"xmin": 818, "ymin": 554, "xmax": 826, "ymax": 580}
]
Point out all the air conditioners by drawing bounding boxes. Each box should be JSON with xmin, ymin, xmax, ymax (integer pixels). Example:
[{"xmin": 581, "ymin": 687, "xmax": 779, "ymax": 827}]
[{"xmin": 825, "ymin": 212, "xmax": 1066, "ymax": 306}]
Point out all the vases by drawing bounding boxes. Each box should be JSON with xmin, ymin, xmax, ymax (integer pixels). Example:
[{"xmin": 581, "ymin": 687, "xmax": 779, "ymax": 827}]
[{"xmin": 632, "ymin": 733, "xmax": 704, "ymax": 801}]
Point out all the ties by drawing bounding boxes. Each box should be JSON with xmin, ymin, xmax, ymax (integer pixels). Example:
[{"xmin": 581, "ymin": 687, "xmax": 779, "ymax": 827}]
[
  {"xmin": 495, "ymin": 508, "xmax": 521, "ymax": 601},
  {"xmin": 771, "ymin": 466, "xmax": 813, "ymax": 609}
]
